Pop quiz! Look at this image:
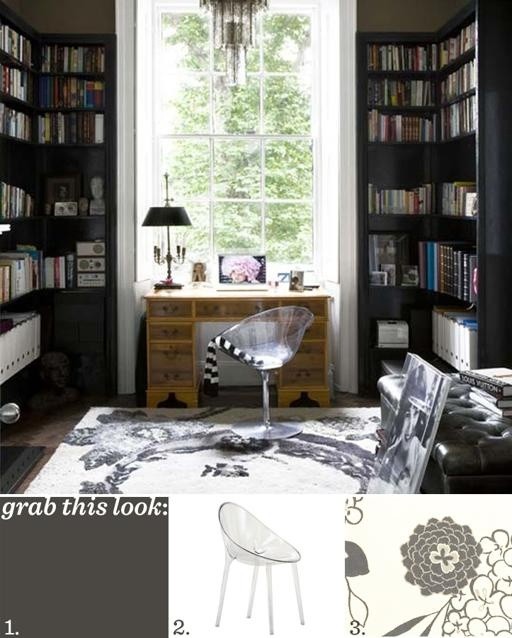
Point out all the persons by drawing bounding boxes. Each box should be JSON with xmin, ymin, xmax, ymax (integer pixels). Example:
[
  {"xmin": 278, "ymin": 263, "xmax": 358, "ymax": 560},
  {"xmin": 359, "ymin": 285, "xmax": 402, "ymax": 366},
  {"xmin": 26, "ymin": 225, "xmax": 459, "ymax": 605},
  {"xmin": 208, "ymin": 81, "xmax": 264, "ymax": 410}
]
[
  {"xmin": 27, "ymin": 351, "xmax": 83, "ymax": 412},
  {"xmin": 192, "ymin": 263, "xmax": 205, "ymax": 282},
  {"xmin": 291, "ymin": 276, "xmax": 299, "ymax": 289},
  {"xmin": 220, "ymin": 257, "xmax": 263, "ymax": 283},
  {"xmin": 375, "ymin": 365, "xmax": 430, "ymax": 488}
]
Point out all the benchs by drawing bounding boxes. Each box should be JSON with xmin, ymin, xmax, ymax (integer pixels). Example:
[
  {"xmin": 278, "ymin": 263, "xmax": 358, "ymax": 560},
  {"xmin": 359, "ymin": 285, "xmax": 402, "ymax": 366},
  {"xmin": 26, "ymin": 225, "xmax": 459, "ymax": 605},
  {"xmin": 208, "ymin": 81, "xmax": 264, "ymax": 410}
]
[{"xmin": 373, "ymin": 372, "xmax": 512, "ymax": 494}]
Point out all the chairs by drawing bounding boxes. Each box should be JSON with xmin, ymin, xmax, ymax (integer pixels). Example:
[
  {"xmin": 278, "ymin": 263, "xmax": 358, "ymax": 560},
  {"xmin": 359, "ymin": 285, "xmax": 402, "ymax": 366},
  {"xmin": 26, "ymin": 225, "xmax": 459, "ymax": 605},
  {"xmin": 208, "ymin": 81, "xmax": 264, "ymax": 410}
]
[
  {"xmin": 214, "ymin": 305, "xmax": 316, "ymax": 441},
  {"xmin": 214, "ymin": 501, "xmax": 306, "ymax": 635}
]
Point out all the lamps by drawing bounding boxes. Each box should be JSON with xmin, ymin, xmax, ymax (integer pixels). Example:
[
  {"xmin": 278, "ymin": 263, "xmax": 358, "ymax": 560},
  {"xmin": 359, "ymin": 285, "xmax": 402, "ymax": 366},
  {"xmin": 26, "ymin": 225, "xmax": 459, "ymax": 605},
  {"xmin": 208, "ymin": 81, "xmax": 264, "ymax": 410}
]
[
  {"xmin": 141, "ymin": 172, "xmax": 193, "ymax": 290},
  {"xmin": 200, "ymin": 0, "xmax": 267, "ymax": 86}
]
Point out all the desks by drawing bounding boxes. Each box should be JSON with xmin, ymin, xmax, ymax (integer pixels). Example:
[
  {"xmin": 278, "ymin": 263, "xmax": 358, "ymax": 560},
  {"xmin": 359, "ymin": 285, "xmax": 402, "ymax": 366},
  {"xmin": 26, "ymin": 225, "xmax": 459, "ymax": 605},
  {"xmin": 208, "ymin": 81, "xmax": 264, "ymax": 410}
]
[{"xmin": 142, "ymin": 287, "xmax": 334, "ymax": 408}]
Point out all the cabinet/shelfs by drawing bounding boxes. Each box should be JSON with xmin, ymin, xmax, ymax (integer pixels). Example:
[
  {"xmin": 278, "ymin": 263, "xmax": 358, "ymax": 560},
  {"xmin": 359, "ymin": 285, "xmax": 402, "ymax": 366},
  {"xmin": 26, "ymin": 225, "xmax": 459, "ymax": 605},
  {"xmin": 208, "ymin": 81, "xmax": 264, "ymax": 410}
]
[
  {"xmin": 356, "ymin": 0, "xmax": 512, "ymax": 395},
  {"xmin": 0, "ymin": 0, "xmax": 119, "ymax": 438}
]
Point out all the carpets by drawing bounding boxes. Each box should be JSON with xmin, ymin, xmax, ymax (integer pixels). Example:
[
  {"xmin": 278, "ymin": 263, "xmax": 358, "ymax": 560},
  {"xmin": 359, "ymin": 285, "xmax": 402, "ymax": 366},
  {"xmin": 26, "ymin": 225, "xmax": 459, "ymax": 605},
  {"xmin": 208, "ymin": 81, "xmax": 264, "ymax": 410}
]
[{"xmin": 22, "ymin": 406, "xmax": 383, "ymax": 496}]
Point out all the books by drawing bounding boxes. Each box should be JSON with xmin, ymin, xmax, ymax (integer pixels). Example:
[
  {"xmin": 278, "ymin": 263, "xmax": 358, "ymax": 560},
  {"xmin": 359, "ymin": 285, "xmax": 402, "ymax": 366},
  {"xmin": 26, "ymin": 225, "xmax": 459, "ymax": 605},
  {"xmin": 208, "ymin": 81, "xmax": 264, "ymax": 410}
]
[
  {"xmin": 371, "ymin": 352, "xmax": 453, "ymax": 493},
  {"xmin": 0, "ymin": 310, "xmax": 45, "ymax": 388},
  {"xmin": 0, "ymin": 22, "xmax": 109, "ymax": 314},
  {"xmin": 466, "ymin": 392, "xmax": 511, "ymax": 419},
  {"xmin": 465, "ymin": 385, "xmax": 511, "ymax": 410},
  {"xmin": 287, "ymin": 270, "xmax": 305, "ymax": 292},
  {"xmin": 458, "ymin": 367, "xmax": 511, "ymax": 398}
]
